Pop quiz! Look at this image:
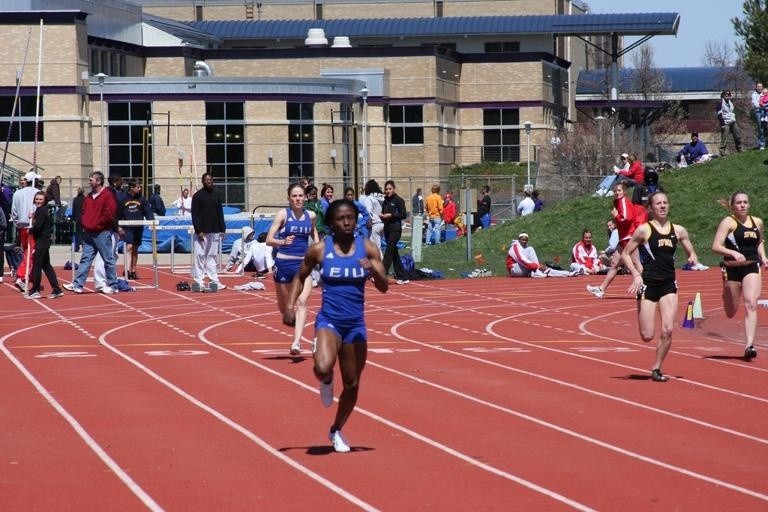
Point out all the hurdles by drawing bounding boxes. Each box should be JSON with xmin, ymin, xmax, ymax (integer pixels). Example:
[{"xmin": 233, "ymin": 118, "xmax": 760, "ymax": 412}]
[{"xmin": 62, "ymin": 200, "xmax": 258, "ymax": 295}]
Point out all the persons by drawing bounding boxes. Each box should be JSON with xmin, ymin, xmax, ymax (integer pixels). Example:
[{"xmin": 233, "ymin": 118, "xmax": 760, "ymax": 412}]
[
  {"xmin": 173, "ymin": 189, "xmax": 192, "ymax": 215},
  {"xmin": 591, "ymin": 152, "xmax": 645, "ymax": 197},
  {"xmin": 300, "ymin": 177, "xmax": 410, "ymax": 285},
  {"xmin": 677, "ymin": 131, "xmax": 709, "ymax": 168},
  {"xmin": 150, "ymin": 184, "xmax": 166, "ymax": 216},
  {"xmin": 586, "ymin": 182, "xmax": 643, "ymax": 300},
  {"xmin": 266, "ymin": 184, "xmax": 320, "ymax": 355},
  {"xmin": 413, "ymin": 184, "xmax": 491, "ymax": 246},
  {"xmin": 621, "ymin": 191, "xmax": 698, "ymax": 382},
  {"xmin": 506, "ymin": 218, "xmax": 620, "ymax": 278},
  {"xmin": 716, "ymin": 88, "xmax": 746, "ymax": 156},
  {"xmin": 633, "ymin": 166, "xmax": 664, "ymax": 207},
  {"xmin": 711, "ymin": 190, "xmax": 768, "ymax": 358},
  {"xmin": 192, "ymin": 172, "xmax": 226, "ymax": 292},
  {"xmin": 751, "ymin": 82, "xmax": 767, "ymax": 151},
  {"xmin": 283, "ymin": 199, "xmax": 388, "ymax": 453},
  {"xmin": 222, "ymin": 226, "xmax": 276, "ymax": 275},
  {"xmin": 515, "ymin": 190, "xmax": 543, "ymax": 217},
  {"xmin": 1, "ymin": 170, "xmax": 156, "ymax": 300}
]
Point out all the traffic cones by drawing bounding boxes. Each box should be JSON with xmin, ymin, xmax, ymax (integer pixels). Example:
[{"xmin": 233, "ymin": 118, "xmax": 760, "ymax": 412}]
[{"xmin": 682, "ymin": 292, "xmax": 706, "ymax": 329}]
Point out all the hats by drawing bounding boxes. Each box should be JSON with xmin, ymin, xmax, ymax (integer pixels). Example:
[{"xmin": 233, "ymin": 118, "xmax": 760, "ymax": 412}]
[
  {"xmin": 443, "ymin": 194, "xmax": 453, "ymax": 198},
  {"xmin": 25, "ymin": 172, "xmax": 41, "ymax": 182},
  {"xmin": 621, "ymin": 153, "xmax": 628, "ymax": 158}
]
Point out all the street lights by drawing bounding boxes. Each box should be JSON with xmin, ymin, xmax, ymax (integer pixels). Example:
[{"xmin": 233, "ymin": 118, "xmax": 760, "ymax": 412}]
[
  {"xmin": 593, "ymin": 115, "xmax": 606, "ymax": 175},
  {"xmin": 93, "ymin": 71, "xmax": 109, "ymax": 176},
  {"xmin": 358, "ymin": 85, "xmax": 370, "ymax": 190},
  {"xmin": 523, "ymin": 120, "xmax": 534, "ymax": 190}
]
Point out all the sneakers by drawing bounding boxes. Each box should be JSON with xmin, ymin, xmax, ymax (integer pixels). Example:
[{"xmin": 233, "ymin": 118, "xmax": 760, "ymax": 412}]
[
  {"xmin": 531, "ymin": 268, "xmax": 547, "ymax": 279},
  {"xmin": 15, "ymin": 279, "xmax": 26, "ymax": 291},
  {"xmin": 744, "ymin": 347, "xmax": 756, "ymax": 361},
  {"xmin": 102, "ymin": 287, "xmax": 118, "ymax": 294},
  {"xmin": 593, "ymin": 189, "xmax": 614, "ymax": 197},
  {"xmin": 587, "ymin": 286, "xmax": 604, "ymax": 299},
  {"xmin": 63, "ymin": 283, "xmax": 81, "ymax": 293},
  {"xmin": 328, "ymin": 427, "xmax": 350, "ymax": 452},
  {"xmin": 128, "ymin": 271, "xmax": 137, "ymax": 280},
  {"xmin": 200, "ymin": 282, "xmax": 227, "ymax": 291},
  {"xmin": 571, "ymin": 268, "xmax": 583, "ymax": 276},
  {"xmin": 652, "ymin": 368, "xmax": 667, "ymax": 382},
  {"xmin": 47, "ymin": 292, "xmax": 63, "ymax": 299},
  {"xmin": 397, "ymin": 279, "xmax": 410, "ymax": 285},
  {"xmin": 290, "ymin": 342, "xmax": 301, "ymax": 356},
  {"xmin": 319, "ymin": 381, "xmax": 335, "ymax": 407},
  {"xmin": 24, "ymin": 292, "xmax": 41, "ymax": 300}
]
